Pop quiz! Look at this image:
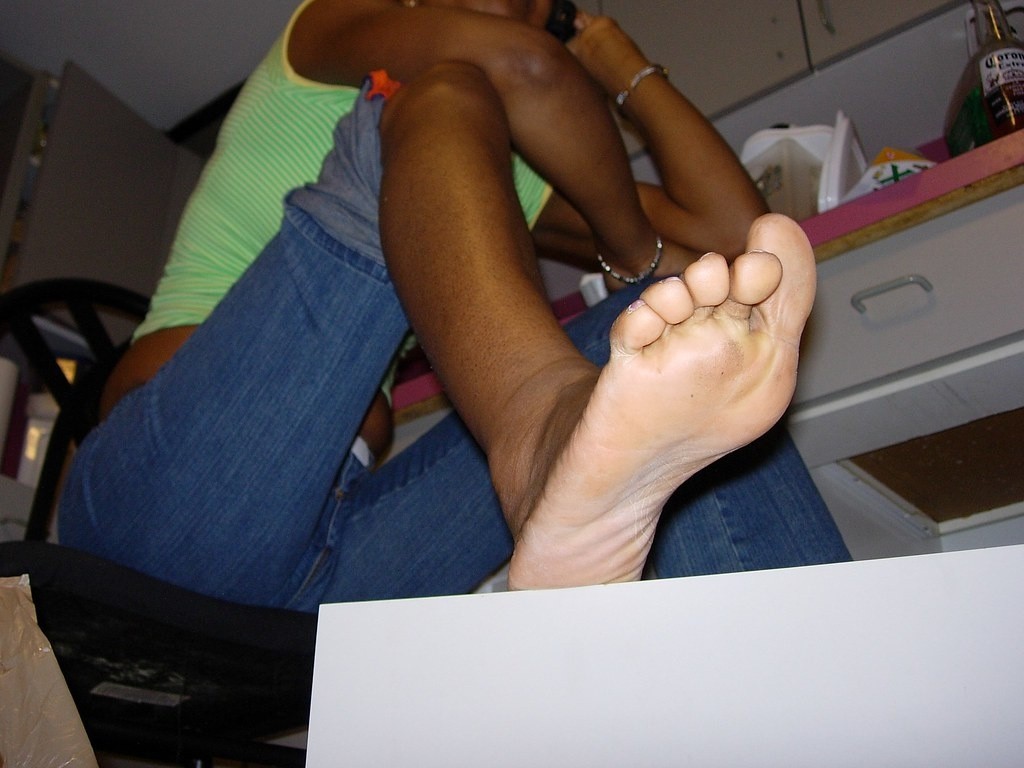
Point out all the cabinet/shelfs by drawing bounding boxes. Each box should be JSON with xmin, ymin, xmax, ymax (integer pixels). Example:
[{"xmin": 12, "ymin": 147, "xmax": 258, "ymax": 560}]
[{"xmin": 386, "ymin": 0, "xmax": 1024, "ymax": 594}]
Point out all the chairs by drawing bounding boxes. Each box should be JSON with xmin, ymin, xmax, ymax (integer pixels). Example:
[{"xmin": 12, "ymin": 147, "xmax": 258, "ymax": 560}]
[{"xmin": 0, "ymin": 275, "xmax": 319, "ymax": 768}]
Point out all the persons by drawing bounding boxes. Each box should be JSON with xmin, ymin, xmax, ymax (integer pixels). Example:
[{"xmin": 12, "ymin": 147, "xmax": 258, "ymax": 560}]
[{"xmin": 57, "ymin": 0, "xmax": 850, "ymax": 615}]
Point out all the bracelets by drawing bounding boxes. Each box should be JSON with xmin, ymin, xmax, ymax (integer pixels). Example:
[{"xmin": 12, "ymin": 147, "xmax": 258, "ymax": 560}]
[
  {"xmin": 616, "ymin": 66, "xmax": 669, "ymax": 115},
  {"xmin": 596, "ymin": 237, "xmax": 662, "ymax": 283}
]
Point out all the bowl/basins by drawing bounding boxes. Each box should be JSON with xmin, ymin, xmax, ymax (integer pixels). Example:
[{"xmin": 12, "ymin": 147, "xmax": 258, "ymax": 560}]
[{"xmin": 744, "ymin": 137, "xmax": 824, "ymax": 221}]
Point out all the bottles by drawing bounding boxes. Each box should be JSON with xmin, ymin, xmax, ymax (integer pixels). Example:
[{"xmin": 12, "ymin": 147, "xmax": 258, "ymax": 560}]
[{"xmin": 972, "ymin": 1, "xmax": 1024, "ymax": 139}]
